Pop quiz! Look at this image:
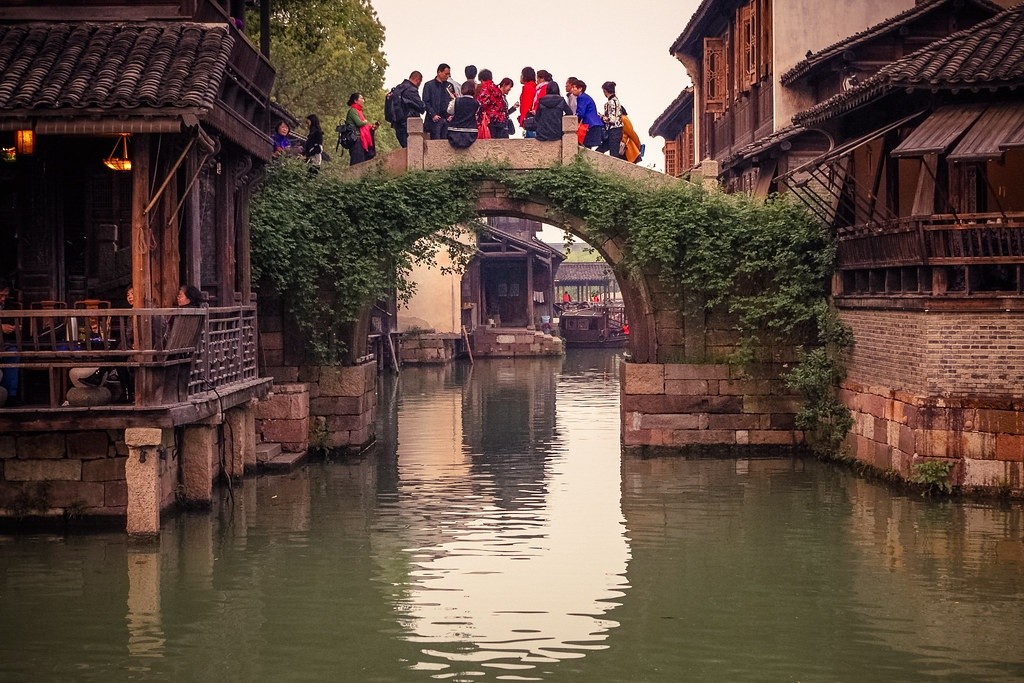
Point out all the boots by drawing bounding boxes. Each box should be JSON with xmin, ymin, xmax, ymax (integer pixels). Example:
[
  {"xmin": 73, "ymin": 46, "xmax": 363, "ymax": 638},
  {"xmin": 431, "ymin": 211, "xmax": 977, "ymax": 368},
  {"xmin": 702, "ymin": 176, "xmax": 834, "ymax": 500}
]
[
  {"xmin": 111, "ymin": 384, "xmax": 133, "ymax": 405},
  {"xmin": 77, "ymin": 368, "xmax": 105, "ymax": 387}
]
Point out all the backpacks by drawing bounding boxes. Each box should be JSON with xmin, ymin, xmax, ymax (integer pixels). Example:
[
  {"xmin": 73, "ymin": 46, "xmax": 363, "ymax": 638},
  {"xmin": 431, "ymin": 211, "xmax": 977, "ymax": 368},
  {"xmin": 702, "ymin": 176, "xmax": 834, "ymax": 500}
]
[
  {"xmin": 384, "ymin": 83, "xmax": 412, "ymax": 128},
  {"xmin": 335, "ymin": 108, "xmax": 358, "ymax": 157}
]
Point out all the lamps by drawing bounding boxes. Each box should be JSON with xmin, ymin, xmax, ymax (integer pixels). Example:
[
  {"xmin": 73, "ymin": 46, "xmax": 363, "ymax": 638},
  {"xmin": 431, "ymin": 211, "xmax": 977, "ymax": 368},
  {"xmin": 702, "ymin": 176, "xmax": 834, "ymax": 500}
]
[
  {"xmin": 102, "ymin": 132, "xmax": 132, "ymax": 171},
  {"xmin": 0, "ymin": 144, "xmax": 16, "ymax": 163},
  {"xmin": 14, "ymin": 129, "xmax": 37, "ymax": 157}
]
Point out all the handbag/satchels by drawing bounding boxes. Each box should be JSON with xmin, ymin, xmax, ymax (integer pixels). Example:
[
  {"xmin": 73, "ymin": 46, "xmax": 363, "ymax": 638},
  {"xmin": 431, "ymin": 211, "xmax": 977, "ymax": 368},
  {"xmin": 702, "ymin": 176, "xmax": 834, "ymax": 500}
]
[
  {"xmin": 576, "ymin": 118, "xmax": 589, "ymax": 145},
  {"xmin": 523, "ymin": 116, "xmax": 536, "ymax": 131},
  {"xmin": 619, "ymin": 136, "xmax": 630, "ymax": 155},
  {"xmin": 508, "ymin": 118, "xmax": 515, "ymax": 135}
]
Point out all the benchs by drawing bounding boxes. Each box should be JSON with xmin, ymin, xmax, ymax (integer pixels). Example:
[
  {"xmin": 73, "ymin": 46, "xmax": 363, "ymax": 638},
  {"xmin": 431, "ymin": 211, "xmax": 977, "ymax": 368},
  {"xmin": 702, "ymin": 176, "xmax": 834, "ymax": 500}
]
[
  {"xmin": 830, "ymin": 212, "xmax": 1024, "ymax": 293},
  {"xmin": 0, "ymin": 304, "xmax": 209, "ymax": 406}
]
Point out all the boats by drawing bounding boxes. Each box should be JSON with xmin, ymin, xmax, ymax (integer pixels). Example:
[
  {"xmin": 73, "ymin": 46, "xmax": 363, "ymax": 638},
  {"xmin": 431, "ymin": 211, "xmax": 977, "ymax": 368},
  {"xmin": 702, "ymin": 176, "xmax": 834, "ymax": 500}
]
[{"xmin": 558, "ymin": 309, "xmax": 630, "ymax": 349}]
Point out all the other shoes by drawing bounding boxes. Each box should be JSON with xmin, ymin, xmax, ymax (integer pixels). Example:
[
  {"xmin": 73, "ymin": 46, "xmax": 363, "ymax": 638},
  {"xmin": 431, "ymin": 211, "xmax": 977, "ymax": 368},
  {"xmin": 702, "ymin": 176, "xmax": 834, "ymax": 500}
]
[{"xmin": 6, "ymin": 396, "xmax": 17, "ymax": 407}]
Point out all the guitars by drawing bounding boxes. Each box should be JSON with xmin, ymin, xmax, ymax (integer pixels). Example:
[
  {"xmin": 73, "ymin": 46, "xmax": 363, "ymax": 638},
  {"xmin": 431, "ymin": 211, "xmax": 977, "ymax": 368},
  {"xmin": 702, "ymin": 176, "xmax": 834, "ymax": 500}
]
[{"xmin": 445, "ymin": 85, "xmax": 456, "ymax": 99}]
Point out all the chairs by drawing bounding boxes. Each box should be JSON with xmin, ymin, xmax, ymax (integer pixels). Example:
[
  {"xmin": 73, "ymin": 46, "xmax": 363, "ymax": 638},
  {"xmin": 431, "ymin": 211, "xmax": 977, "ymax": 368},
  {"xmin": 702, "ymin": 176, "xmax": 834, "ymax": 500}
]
[
  {"xmin": 31, "ymin": 300, "xmax": 67, "ymax": 342},
  {"xmin": 74, "ymin": 298, "xmax": 116, "ymax": 349}
]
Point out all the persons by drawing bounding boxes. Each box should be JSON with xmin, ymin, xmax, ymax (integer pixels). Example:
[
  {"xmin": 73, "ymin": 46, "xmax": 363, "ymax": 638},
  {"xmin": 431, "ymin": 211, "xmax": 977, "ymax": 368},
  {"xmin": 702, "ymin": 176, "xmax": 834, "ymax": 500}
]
[
  {"xmin": 106, "ymin": 285, "xmax": 167, "ymax": 385},
  {"xmin": 0, "ymin": 283, "xmax": 22, "ymax": 408},
  {"xmin": 394, "ymin": 62, "xmax": 643, "ymax": 165},
  {"xmin": 77, "ymin": 285, "xmax": 203, "ymax": 405},
  {"xmin": 563, "ymin": 291, "xmax": 571, "ymax": 303},
  {"xmin": 345, "ymin": 93, "xmax": 379, "ymax": 166},
  {"xmin": 295, "ymin": 114, "xmax": 324, "ymax": 177},
  {"xmin": 269, "ymin": 120, "xmax": 291, "ymax": 160}
]
